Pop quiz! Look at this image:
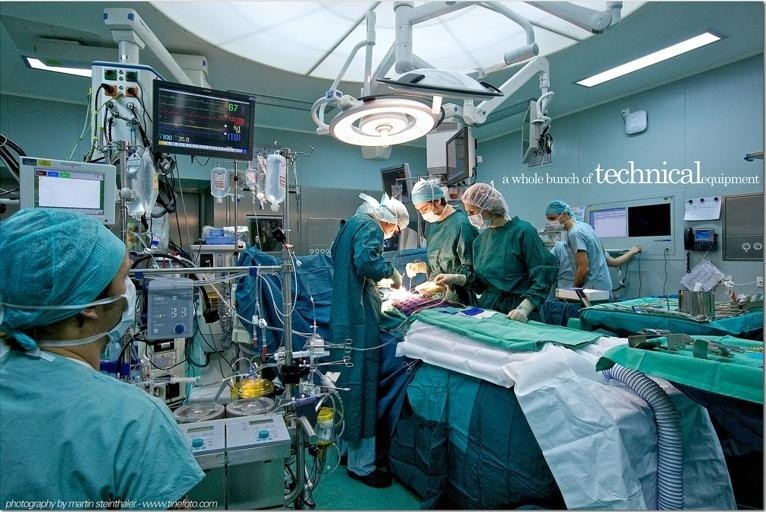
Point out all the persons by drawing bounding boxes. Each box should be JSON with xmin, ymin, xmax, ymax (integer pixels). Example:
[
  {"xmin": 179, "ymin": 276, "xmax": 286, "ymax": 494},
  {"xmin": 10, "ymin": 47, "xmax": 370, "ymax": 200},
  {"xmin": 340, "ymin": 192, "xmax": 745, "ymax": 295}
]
[
  {"xmin": 0, "ymin": 207, "xmax": 207, "ymax": 510},
  {"xmin": 329, "ymin": 196, "xmax": 410, "ymax": 489},
  {"xmin": 411, "ymin": 179, "xmax": 642, "ymax": 322}
]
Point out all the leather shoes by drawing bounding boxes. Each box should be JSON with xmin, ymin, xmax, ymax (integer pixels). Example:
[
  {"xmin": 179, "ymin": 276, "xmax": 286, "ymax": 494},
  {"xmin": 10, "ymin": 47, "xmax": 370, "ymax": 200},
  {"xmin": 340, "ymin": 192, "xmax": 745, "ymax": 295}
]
[{"xmin": 347, "ymin": 467, "xmax": 392, "ymax": 486}]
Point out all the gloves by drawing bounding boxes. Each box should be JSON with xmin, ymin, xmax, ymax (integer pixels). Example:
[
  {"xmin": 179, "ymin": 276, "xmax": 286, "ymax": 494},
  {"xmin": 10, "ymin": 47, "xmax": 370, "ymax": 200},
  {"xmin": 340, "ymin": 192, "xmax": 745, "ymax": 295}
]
[
  {"xmin": 406, "ymin": 262, "xmax": 427, "ymax": 278},
  {"xmin": 507, "ymin": 298, "xmax": 535, "ymax": 322},
  {"xmin": 414, "ymin": 273, "xmax": 466, "ymax": 298}
]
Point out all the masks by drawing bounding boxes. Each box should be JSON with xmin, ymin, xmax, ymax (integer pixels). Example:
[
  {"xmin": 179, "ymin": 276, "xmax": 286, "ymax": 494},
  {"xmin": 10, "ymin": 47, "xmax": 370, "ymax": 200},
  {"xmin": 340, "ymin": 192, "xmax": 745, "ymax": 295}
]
[
  {"xmin": 549, "ymin": 215, "xmax": 569, "ymax": 229},
  {"xmin": 468, "ymin": 209, "xmax": 493, "ymax": 230},
  {"xmin": 421, "ymin": 204, "xmax": 444, "ymax": 223},
  {"xmin": 98, "ymin": 275, "xmax": 136, "ymax": 343},
  {"xmin": 380, "ymin": 224, "xmax": 397, "ymax": 240}
]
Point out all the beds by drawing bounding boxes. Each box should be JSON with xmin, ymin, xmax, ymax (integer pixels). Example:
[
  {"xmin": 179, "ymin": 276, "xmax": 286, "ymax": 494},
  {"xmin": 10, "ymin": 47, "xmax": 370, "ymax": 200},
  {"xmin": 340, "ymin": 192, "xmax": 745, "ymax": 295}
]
[{"xmin": 310, "ymin": 284, "xmax": 635, "ymax": 507}]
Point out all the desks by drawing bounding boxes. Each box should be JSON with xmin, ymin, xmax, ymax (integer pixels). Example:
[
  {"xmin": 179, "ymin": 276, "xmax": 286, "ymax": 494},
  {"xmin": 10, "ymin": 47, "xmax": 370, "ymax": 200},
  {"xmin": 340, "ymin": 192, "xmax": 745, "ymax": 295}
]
[{"xmin": 576, "ymin": 296, "xmax": 766, "ymax": 409}]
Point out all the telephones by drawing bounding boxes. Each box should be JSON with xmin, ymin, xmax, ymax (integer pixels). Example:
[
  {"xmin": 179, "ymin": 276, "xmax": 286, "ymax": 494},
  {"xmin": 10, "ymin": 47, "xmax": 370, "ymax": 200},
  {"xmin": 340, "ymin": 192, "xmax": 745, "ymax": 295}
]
[{"xmin": 684, "ymin": 228, "xmax": 716, "ymax": 250}]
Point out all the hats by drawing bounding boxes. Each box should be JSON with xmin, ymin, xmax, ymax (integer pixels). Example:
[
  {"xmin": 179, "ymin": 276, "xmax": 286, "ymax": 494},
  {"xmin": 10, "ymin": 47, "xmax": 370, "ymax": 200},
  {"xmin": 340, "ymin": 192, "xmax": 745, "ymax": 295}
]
[
  {"xmin": 545, "ymin": 200, "xmax": 575, "ymax": 216},
  {"xmin": 379, "ymin": 199, "xmax": 410, "ymax": 231},
  {"xmin": 411, "ymin": 180, "xmax": 444, "ymax": 205},
  {"xmin": 0, "ymin": 208, "xmax": 126, "ymax": 328}
]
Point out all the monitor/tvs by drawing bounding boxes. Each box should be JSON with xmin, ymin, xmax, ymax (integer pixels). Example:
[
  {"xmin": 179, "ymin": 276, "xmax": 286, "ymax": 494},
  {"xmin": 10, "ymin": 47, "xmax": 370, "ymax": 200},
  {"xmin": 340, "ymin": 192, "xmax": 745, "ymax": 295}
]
[
  {"xmin": 152, "ymin": 79, "xmax": 255, "ymax": 161},
  {"xmin": 380, "ymin": 163, "xmax": 411, "ymax": 203},
  {"xmin": 446, "ymin": 126, "xmax": 469, "ymax": 186},
  {"xmin": 20, "ymin": 156, "xmax": 116, "ymax": 225},
  {"xmin": 589, "ymin": 207, "xmax": 626, "ymax": 239},
  {"xmin": 626, "ymin": 203, "xmax": 672, "ymax": 237},
  {"xmin": 522, "ymin": 100, "xmax": 534, "ymax": 163}
]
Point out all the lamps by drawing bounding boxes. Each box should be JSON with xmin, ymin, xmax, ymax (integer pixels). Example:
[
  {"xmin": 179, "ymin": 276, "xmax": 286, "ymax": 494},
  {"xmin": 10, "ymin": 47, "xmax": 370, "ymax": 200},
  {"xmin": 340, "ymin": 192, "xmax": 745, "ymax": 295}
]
[{"xmin": 292, "ymin": 0, "xmax": 556, "ymax": 169}]
